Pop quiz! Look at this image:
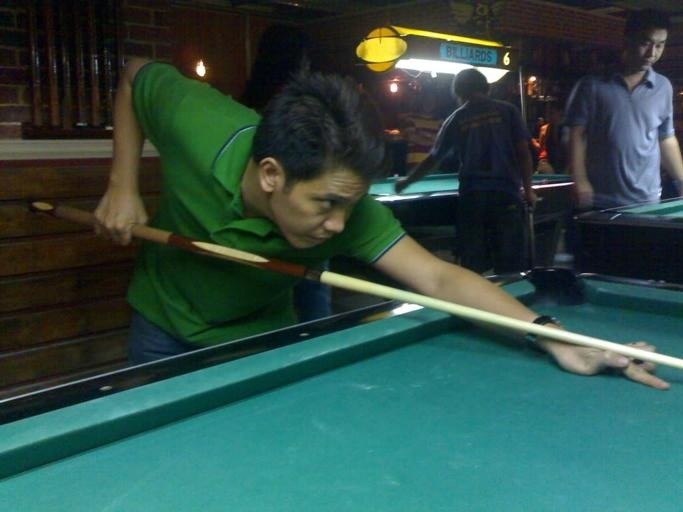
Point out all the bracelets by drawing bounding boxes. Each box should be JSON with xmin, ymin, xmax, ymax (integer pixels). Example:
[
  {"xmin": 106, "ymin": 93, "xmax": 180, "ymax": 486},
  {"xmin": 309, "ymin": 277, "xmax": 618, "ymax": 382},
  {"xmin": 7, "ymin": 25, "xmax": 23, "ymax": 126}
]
[{"xmin": 523, "ymin": 314, "xmax": 563, "ymax": 357}]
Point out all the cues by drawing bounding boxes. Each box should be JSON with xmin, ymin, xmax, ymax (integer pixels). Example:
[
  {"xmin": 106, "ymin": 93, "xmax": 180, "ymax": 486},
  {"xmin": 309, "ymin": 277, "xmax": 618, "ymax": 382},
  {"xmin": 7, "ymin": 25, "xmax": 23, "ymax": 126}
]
[
  {"xmin": 519, "ymin": 66, "xmax": 534, "ymax": 268},
  {"xmin": 26, "ymin": 0, "xmax": 124, "ymax": 129},
  {"xmin": 29, "ymin": 202, "xmax": 683, "ymax": 369}
]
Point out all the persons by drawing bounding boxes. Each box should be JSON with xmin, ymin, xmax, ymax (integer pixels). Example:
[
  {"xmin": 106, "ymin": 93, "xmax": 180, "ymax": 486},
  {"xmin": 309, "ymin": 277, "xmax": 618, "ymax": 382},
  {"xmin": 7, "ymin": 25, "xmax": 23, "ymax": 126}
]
[
  {"xmin": 393, "ymin": 69, "xmax": 537, "ymax": 276},
  {"xmin": 560, "ymin": 7, "xmax": 683, "ymax": 212},
  {"xmin": 91, "ymin": 56, "xmax": 675, "ymax": 392},
  {"xmin": 530, "ymin": 94, "xmax": 566, "ymax": 176},
  {"xmin": 235, "ymin": 11, "xmax": 334, "ymax": 323},
  {"xmin": 341, "ymin": 70, "xmax": 407, "ymax": 281}
]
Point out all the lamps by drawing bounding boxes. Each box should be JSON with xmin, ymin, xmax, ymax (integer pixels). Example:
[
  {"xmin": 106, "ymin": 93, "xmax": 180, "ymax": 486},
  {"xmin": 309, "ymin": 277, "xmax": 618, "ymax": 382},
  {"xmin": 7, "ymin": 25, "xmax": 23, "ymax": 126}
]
[{"xmin": 350, "ymin": 25, "xmax": 410, "ymax": 66}]
